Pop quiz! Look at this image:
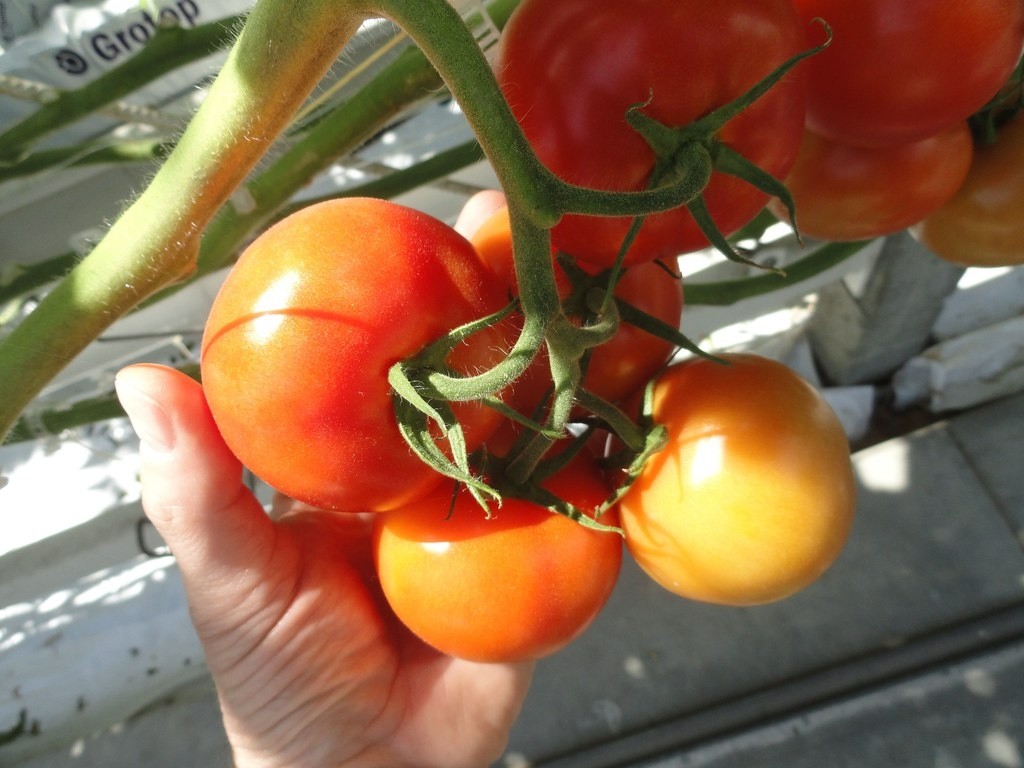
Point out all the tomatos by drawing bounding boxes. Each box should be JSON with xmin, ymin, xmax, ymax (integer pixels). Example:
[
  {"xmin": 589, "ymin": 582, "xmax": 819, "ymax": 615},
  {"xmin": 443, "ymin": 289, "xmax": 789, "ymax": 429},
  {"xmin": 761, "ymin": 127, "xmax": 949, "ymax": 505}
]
[{"xmin": 198, "ymin": 1, "xmax": 1024, "ymax": 661}]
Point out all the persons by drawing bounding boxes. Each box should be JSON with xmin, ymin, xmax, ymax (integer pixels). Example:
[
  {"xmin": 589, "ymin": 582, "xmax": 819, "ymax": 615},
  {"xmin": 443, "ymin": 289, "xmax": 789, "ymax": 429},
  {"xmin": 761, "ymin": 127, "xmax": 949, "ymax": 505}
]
[{"xmin": 114, "ymin": 357, "xmax": 536, "ymax": 768}]
